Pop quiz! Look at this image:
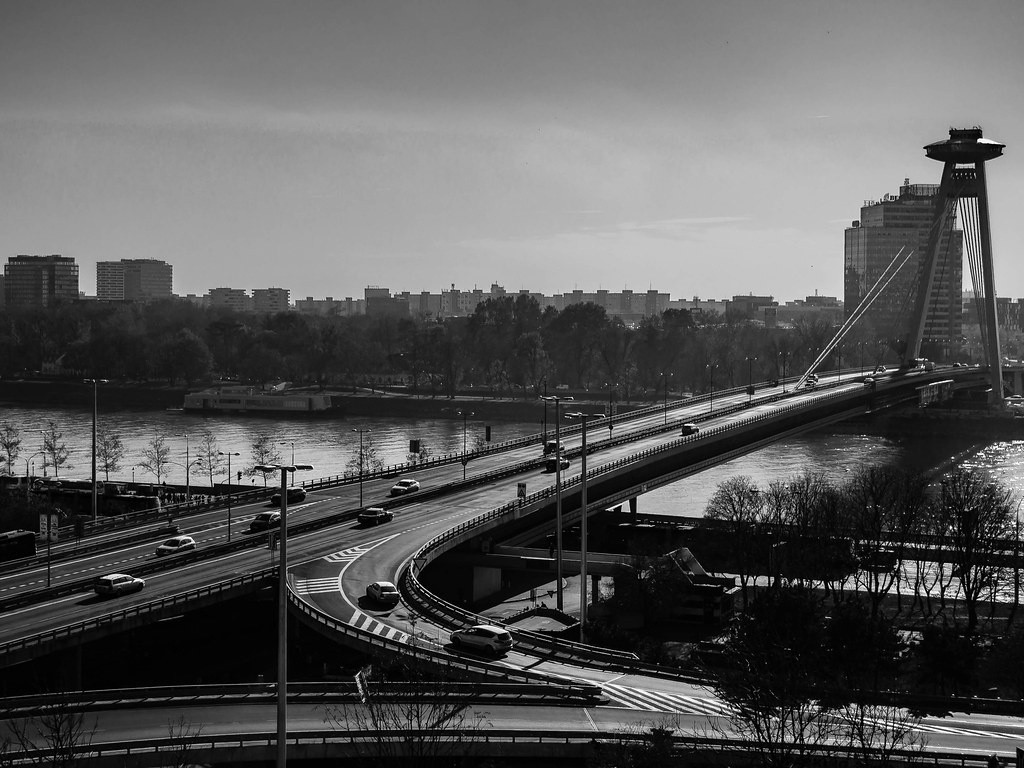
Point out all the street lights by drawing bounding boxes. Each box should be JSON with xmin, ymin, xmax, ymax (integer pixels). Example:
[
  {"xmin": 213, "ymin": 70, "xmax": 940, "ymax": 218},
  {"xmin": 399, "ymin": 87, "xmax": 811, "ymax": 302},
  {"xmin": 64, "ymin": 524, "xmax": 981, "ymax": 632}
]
[
  {"xmin": 564, "ymin": 411, "xmax": 606, "ymax": 643},
  {"xmin": 218, "ymin": 451, "xmax": 240, "ymax": 541},
  {"xmin": 280, "ymin": 442, "xmax": 295, "ymax": 486},
  {"xmin": 540, "ymin": 396, "xmax": 574, "ymax": 610},
  {"xmin": 779, "ymin": 351, "xmax": 790, "ymax": 392},
  {"xmin": 11, "ymin": 451, "xmax": 45, "ymax": 490},
  {"xmin": 352, "ymin": 428, "xmax": 371, "ymax": 507},
  {"xmin": 255, "ymin": 464, "xmax": 315, "ymax": 768},
  {"xmin": 809, "ymin": 347, "xmax": 819, "ymax": 386},
  {"xmin": 834, "ymin": 338, "xmax": 1011, "ymax": 385},
  {"xmin": 660, "ymin": 372, "xmax": 673, "ymax": 424},
  {"xmin": 457, "ymin": 411, "xmax": 474, "ymax": 479},
  {"xmin": 744, "ymin": 357, "xmax": 758, "ymax": 400},
  {"xmin": 706, "ymin": 364, "xmax": 718, "ymax": 412},
  {"xmin": 82, "ymin": 378, "xmax": 109, "ymax": 521},
  {"xmin": 604, "ymin": 383, "xmax": 618, "ymax": 438}
]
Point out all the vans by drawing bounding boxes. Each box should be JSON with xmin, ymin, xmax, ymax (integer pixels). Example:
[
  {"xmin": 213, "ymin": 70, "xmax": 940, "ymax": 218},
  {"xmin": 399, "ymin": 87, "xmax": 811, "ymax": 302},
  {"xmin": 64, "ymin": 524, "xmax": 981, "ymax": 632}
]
[
  {"xmin": 95, "ymin": 573, "xmax": 146, "ymax": 598},
  {"xmin": 860, "ymin": 550, "xmax": 897, "ymax": 568}
]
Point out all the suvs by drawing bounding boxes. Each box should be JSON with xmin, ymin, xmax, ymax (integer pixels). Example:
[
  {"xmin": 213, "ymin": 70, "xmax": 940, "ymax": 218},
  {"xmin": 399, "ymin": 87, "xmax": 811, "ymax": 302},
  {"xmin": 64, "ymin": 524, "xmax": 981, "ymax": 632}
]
[
  {"xmin": 682, "ymin": 424, "xmax": 700, "ymax": 436},
  {"xmin": 545, "ymin": 457, "xmax": 570, "ymax": 472},
  {"xmin": 450, "ymin": 624, "xmax": 514, "ymax": 659},
  {"xmin": 271, "ymin": 487, "xmax": 306, "ymax": 507}
]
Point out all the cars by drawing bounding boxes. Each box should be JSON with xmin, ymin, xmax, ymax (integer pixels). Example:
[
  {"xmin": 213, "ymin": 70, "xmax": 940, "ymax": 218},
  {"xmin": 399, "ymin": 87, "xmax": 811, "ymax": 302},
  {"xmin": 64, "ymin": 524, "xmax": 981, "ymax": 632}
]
[
  {"xmin": 155, "ymin": 536, "xmax": 197, "ymax": 558},
  {"xmin": 358, "ymin": 507, "xmax": 394, "ymax": 527},
  {"xmin": 390, "ymin": 478, "xmax": 420, "ymax": 496},
  {"xmin": 366, "ymin": 581, "xmax": 401, "ymax": 606},
  {"xmin": 250, "ymin": 511, "xmax": 282, "ymax": 533},
  {"xmin": 543, "ymin": 441, "xmax": 565, "ymax": 455},
  {"xmin": 805, "ymin": 374, "xmax": 819, "ymax": 387}
]
